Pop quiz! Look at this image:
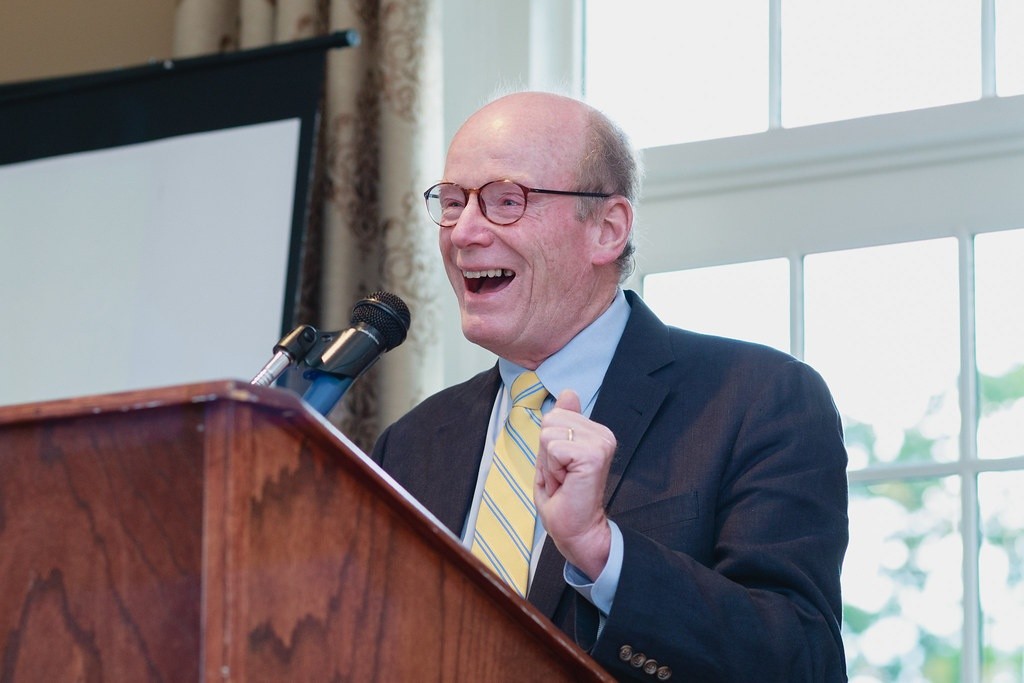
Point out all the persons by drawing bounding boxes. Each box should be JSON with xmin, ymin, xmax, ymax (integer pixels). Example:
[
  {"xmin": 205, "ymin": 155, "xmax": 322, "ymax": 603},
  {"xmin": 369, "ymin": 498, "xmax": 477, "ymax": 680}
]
[{"xmin": 365, "ymin": 83, "xmax": 852, "ymax": 683}]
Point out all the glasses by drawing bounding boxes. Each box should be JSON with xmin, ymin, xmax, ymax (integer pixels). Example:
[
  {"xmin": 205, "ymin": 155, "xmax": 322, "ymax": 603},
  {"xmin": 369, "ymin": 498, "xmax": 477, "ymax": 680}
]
[{"xmin": 424, "ymin": 179, "xmax": 615, "ymax": 228}]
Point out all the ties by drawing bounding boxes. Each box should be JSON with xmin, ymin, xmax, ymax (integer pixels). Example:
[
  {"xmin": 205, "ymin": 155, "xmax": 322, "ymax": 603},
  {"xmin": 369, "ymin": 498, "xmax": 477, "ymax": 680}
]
[{"xmin": 471, "ymin": 370, "xmax": 550, "ymax": 602}]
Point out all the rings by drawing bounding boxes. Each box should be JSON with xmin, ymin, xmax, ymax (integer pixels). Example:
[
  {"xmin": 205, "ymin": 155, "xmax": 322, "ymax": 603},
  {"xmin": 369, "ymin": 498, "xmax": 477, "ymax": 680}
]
[{"xmin": 568, "ymin": 427, "xmax": 575, "ymax": 441}]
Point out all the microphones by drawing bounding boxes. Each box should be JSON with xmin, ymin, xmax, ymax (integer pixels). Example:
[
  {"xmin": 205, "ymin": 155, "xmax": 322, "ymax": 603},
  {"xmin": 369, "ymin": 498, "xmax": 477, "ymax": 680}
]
[{"xmin": 302, "ymin": 290, "xmax": 411, "ymax": 417}]
